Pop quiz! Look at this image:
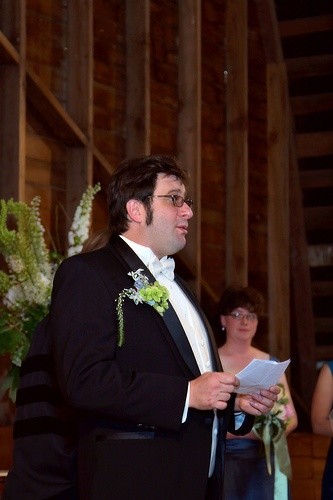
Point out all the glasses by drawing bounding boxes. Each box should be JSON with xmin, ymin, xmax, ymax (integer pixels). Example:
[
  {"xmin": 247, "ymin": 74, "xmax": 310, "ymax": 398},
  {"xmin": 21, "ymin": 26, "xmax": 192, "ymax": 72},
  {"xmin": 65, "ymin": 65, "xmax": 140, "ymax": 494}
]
[
  {"xmin": 147, "ymin": 194, "xmax": 192, "ymax": 209},
  {"xmin": 225, "ymin": 311, "xmax": 257, "ymax": 320}
]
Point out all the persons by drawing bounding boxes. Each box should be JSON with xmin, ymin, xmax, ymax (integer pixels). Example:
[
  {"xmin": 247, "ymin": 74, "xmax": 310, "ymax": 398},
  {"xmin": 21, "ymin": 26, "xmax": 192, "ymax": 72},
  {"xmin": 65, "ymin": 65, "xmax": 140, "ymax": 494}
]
[
  {"xmin": 216, "ymin": 285, "xmax": 298, "ymax": 500},
  {"xmin": 50, "ymin": 153, "xmax": 280, "ymax": 500},
  {"xmin": 310, "ymin": 360, "xmax": 333, "ymax": 500},
  {"xmin": 3, "ymin": 228, "xmax": 111, "ymax": 500}
]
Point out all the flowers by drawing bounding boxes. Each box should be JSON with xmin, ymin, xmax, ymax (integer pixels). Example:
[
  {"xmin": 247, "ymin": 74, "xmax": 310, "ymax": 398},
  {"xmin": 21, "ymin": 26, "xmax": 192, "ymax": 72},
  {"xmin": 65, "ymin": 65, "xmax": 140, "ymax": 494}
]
[
  {"xmin": 0, "ymin": 180, "xmax": 102, "ymax": 403},
  {"xmin": 117, "ymin": 269, "xmax": 169, "ymax": 348},
  {"xmin": 252, "ymin": 382, "xmax": 296, "ymax": 479}
]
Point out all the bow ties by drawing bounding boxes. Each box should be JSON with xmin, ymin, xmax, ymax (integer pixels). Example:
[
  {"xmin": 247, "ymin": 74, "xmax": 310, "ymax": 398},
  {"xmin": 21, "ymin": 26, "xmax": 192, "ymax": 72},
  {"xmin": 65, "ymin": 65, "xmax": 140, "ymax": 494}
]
[{"xmin": 149, "ymin": 257, "xmax": 175, "ymax": 281}]
